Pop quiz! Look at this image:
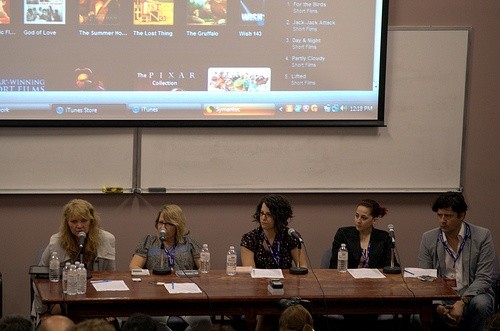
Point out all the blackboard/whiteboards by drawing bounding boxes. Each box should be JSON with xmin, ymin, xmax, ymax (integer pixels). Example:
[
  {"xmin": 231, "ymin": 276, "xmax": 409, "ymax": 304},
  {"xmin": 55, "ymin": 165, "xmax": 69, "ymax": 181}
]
[{"xmin": 0, "ymin": 25, "xmax": 474, "ymax": 197}]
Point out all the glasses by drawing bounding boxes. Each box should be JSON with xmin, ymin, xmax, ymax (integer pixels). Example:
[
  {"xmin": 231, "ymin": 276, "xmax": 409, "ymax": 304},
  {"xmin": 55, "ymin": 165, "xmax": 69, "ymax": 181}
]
[
  {"xmin": 260, "ymin": 211, "xmax": 273, "ymax": 217},
  {"xmin": 157, "ymin": 220, "xmax": 175, "ymax": 227}
]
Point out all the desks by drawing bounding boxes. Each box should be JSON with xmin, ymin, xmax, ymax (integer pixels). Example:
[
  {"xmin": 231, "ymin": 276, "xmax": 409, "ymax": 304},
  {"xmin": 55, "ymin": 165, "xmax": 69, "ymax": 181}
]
[{"xmin": 30, "ymin": 267, "xmax": 461, "ymax": 331}]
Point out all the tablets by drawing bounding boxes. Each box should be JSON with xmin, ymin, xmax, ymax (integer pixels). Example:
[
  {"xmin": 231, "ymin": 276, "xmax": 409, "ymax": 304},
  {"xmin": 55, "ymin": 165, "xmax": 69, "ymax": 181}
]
[{"xmin": 28, "ymin": 265, "xmax": 62, "ymax": 276}]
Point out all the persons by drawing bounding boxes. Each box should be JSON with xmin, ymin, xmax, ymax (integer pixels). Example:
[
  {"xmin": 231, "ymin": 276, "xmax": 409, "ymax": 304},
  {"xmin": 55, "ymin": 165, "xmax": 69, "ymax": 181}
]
[
  {"xmin": 120, "ymin": 313, "xmax": 170, "ymax": 331},
  {"xmin": 74, "ymin": 68, "xmax": 93, "ymax": 91},
  {"xmin": 65, "ymin": 319, "xmax": 115, "ymax": 331},
  {"xmin": 328, "ymin": 199, "xmax": 400, "ymax": 331},
  {"xmin": 240, "ymin": 194, "xmax": 308, "ymax": 331},
  {"xmin": 0, "ymin": 314, "xmax": 34, "ymax": 331},
  {"xmin": 31, "ymin": 198, "xmax": 120, "ymax": 331},
  {"xmin": 35, "ymin": 315, "xmax": 75, "ymax": 331},
  {"xmin": 129, "ymin": 204, "xmax": 212, "ymax": 331},
  {"xmin": 279, "ymin": 304, "xmax": 315, "ymax": 331},
  {"xmin": 418, "ymin": 192, "xmax": 495, "ymax": 331}
]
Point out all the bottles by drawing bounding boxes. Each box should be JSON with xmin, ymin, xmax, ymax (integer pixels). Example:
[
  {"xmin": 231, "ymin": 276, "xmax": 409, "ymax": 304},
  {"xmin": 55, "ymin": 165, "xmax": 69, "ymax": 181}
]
[
  {"xmin": 200, "ymin": 244, "xmax": 210, "ymax": 274},
  {"xmin": 76, "ymin": 264, "xmax": 87, "ymax": 294},
  {"xmin": 49, "ymin": 252, "xmax": 60, "ymax": 282},
  {"xmin": 67, "ymin": 265, "xmax": 77, "ymax": 295},
  {"xmin": 63, "ymin": 262, "xmax": 71, "ymax": 293},
  {"xmin": 75, "ymin": 262, "xmax": 80, "ymax": 271},
  {"xmin": 337, "ymin": 244, "xmax": 348, "ymax": 272},
  {"xmin": 227, "ymin": 246, "xmax": 237, "ymax": 276}
]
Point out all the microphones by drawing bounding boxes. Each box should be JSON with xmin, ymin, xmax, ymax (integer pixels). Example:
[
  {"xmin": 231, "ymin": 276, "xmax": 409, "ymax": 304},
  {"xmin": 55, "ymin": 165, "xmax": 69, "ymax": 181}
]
[
  {"xmin": 388, "ymin": 223, "xmax": 395, "ymax": 244},
  {"xmin": 288, "ymin": 228, "xmax": 302, "ymax": 242},
  {"xmin": 160, "ymin": 228, "xmax": 166, "ymax": 240},
  {"xmin": 78, "ymin": 232, "xmax": 86, "ymax": 247}
]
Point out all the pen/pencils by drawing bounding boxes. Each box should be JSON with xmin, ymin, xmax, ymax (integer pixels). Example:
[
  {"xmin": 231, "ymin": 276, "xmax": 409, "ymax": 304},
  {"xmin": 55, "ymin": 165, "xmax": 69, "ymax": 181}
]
[
  {"xmin": 403, "ymin": 269, "xmax": 414, "ymax": 275},
  {"xmin": 87, "ymin": 279, "xmax": 112, "ymax": 284},
  {"xmin": 172, "ymin": 282, "xmax": 174, "ymax": 289}
]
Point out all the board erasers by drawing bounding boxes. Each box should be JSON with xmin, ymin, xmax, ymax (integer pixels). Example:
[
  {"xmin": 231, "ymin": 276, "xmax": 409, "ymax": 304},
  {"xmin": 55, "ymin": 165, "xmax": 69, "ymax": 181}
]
[
  {"xmin": 105, "ymin": 188, "xmax": 122, "ymax": 192},
  {"xmin": 148, "ymin": 187, "xmax": 167, "ymax": 192}
]
